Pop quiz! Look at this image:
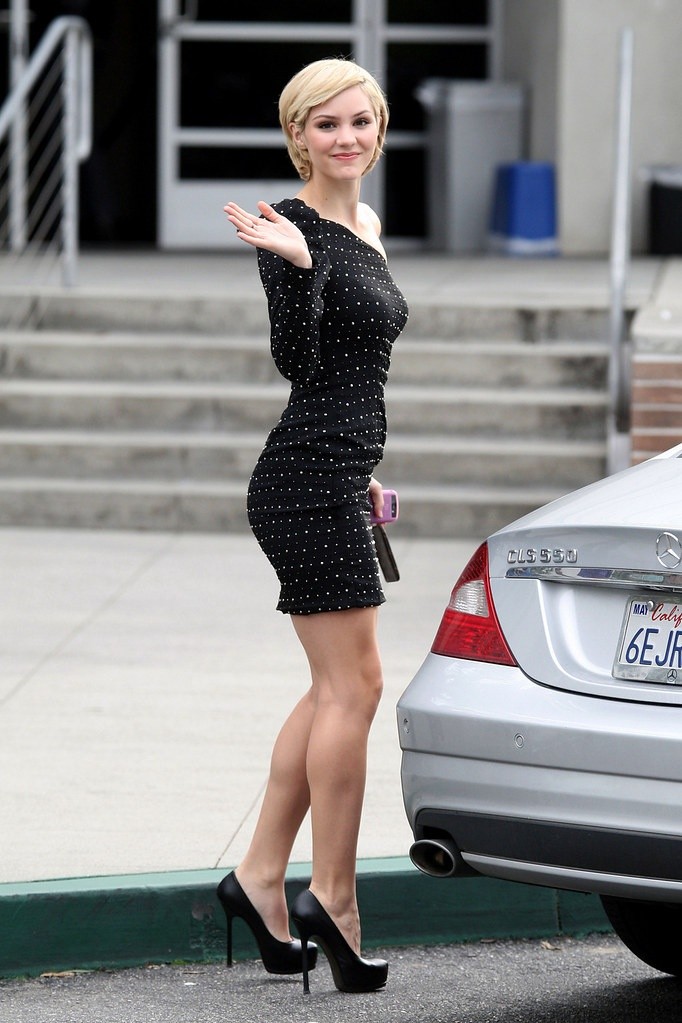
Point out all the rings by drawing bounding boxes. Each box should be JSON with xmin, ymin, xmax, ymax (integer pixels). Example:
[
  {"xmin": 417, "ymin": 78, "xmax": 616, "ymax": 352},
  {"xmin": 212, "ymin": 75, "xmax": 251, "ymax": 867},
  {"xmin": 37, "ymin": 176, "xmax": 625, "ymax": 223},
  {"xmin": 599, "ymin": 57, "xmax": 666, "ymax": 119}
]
[{"xmin": 252, "ymin": 225, "xmax": 254, "ymax": 228}]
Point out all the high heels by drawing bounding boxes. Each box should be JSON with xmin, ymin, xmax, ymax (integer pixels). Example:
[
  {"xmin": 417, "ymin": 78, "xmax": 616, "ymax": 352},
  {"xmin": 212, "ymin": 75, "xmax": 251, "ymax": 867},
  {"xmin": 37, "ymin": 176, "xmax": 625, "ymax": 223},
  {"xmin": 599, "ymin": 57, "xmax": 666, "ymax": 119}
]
[
  {"xmin": 216, "ymin": 870, "xmax": 318, "ymax": 975},
  {"xmin": 289, "ymin": 889, "xmax": 389, "ymax": 994}
]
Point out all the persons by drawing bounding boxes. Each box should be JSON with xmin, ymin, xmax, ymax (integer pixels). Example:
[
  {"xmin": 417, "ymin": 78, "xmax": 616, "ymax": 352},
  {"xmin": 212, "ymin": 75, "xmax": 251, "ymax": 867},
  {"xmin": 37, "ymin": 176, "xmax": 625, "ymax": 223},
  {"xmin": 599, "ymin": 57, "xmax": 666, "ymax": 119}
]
[{"xmin": 215, "ymin": 58, "xmax": 411, "ymax": 994}]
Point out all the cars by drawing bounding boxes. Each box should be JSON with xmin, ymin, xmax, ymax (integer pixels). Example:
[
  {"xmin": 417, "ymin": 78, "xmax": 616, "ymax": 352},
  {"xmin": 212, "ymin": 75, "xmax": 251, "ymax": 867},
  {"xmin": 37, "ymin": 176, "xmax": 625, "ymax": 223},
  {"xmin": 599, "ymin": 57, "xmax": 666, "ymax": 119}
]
[{"xmin": 397, "ymin": 443, "xmax": 682, "ymax": 977}]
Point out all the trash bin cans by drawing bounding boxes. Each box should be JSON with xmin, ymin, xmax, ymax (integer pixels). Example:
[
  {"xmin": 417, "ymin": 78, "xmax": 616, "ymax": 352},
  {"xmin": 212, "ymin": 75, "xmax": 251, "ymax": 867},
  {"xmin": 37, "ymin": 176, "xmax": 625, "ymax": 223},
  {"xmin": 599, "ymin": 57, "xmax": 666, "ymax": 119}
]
[
  {"xmin": 416, "ymin": 81, "xmax": 525, "ymax": 253},
  {"xmin": 643, "ymin": 166, "xmax": 682, "ymax": 257}
]
